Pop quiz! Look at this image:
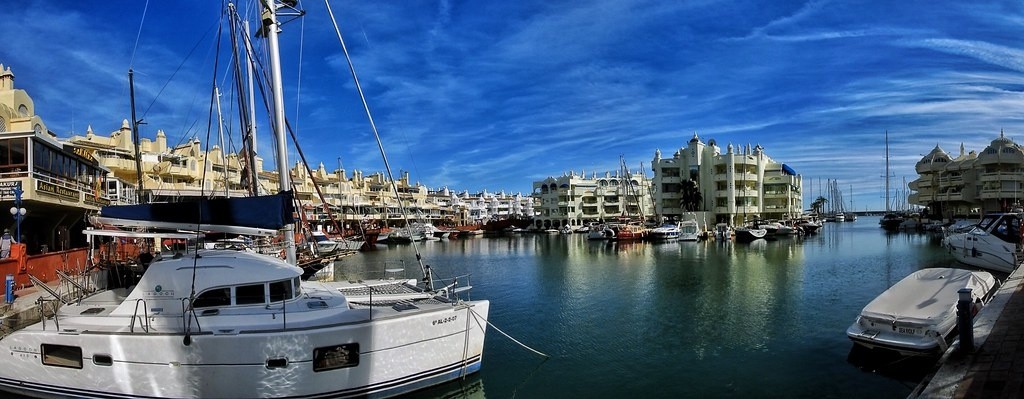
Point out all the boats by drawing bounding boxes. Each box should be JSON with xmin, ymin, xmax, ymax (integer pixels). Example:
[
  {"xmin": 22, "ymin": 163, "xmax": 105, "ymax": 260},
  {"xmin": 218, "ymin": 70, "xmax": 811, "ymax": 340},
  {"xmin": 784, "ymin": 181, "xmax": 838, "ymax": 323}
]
[
  {"xmin": 880, "ymin": 176, "xmax": 1024, "ymax": 273},
  {"xmin": 846, "ymin": 263, "xmax": 997, "ymax": 358}
]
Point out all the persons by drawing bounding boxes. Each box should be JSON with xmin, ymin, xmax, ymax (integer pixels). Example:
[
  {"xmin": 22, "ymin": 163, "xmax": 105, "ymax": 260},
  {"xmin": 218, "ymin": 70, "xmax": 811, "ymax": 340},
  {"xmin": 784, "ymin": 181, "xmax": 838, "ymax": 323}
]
[{"xmin": 0, "ymin": 228, "xmax": 18, "ymax": 258}]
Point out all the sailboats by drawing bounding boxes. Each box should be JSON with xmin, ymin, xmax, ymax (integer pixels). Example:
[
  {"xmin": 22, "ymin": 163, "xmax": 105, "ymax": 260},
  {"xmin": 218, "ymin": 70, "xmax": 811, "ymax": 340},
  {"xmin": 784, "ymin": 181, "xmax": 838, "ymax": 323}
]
[{"xmin": 0, "ymin": 0, "xmax": 863, "ymax": 399}]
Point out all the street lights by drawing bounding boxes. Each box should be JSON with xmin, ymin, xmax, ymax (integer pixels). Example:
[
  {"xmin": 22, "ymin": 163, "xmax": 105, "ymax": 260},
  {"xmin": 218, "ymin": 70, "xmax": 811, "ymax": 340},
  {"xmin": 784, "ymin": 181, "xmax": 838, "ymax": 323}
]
[{"xmin": 10, "ymin": 208, "xmax": 27, "ymax": 245}]
